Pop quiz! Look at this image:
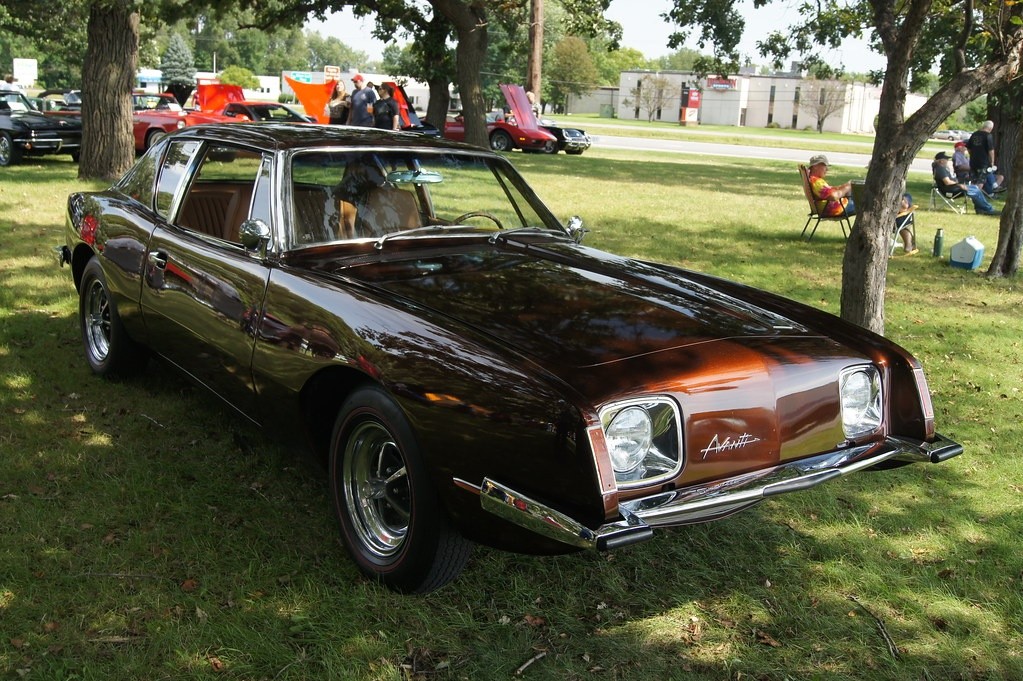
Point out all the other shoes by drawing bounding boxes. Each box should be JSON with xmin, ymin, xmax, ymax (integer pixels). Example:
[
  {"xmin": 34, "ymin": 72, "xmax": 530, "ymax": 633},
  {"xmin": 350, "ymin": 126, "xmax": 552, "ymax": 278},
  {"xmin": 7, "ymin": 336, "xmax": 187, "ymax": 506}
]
[
  {"xmin": 904, "ymin": 247, "xmax": 913, "ymax": 252},
  {"xmin": 993, "ymin": 186, "xmax": 1007, "ymax": 193},
  {"xmin": 976, "ymin": 210, "xmax": 987, "ymax": 214},
  {"xmin": 989, "ymin": 211, "xmax": 1001, "ymax": 216}
]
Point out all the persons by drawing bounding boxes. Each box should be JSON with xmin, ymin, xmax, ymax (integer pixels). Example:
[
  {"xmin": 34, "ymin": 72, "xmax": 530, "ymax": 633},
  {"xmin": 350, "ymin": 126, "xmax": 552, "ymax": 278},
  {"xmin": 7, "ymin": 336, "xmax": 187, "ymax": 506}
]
[
  {"xmin": 503, "ymin": 100, "xmax": 515, "ymax": 123},
  {"xmin": 192, "ymin": 89, "xmax": 200, "ymax": 110},
  {"xmin": 807, "ymin": 154, "xmax": 855, "ymax": 217},
  {"xmin": 895, "ymin": 197, "xmax": 913, "ymax": 251},
  {"xmin": 953, "ymin": 122, "xmax": 1007, "ymax": 200},
  {"xmin": 328, "ymin": 75, "xmax": 400, "ymax": 171},
  {"xmin": 526, "ymin": 92, "xmax": 541, "ymax": 119},
  {"xmin": 934, "ymin": 151, "xmax": 1002, "ymax": 215},
  {"xmin": 3, "ymin": 75, "xmax": 19, "ymax": 102}
]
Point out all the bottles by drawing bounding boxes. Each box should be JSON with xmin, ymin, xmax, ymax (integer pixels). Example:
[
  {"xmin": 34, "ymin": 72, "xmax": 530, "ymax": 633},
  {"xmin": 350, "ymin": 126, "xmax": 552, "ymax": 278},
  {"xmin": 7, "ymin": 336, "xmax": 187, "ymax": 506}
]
[{"xmin": 934, "ymin": 228, "xmax": 942, "ymax": 257}]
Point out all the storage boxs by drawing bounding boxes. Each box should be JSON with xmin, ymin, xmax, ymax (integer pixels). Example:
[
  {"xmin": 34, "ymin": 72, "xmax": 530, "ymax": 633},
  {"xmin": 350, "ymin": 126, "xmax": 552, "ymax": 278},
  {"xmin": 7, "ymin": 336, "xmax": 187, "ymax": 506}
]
[{"xmin": 950, "ymin": 236, "xmax": 984, "ymax": 269}]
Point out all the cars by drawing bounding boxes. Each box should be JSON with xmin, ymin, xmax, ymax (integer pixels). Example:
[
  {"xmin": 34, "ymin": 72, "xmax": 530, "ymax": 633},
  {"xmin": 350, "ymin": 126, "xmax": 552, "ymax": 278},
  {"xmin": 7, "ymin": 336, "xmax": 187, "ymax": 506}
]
[
  {"xmin": 438, "ymin": 84, "xmax": 593, "ymax": 156},
  {"xmin": 33, "ymin": 74, "xmax": 441, "ymax": 164},
  {"xmin": 57, "ymin": 118, "xmax": 965, "ymax": 594},
  {"xmin": 0, "ymin": 80, "xmax": 85, "ymax": 168}
]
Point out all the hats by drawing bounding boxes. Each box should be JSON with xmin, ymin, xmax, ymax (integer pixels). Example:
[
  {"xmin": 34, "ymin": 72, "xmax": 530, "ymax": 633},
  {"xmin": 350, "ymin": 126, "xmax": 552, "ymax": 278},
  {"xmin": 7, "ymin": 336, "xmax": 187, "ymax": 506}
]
[
  {"xmin": 807, "ymin": 154, "xmax": 832, "ymax": 169},
  {"xmin": 935, "ymin": 152, "xmax": 952, "ymax": 159},
  {"xmin": 351, "ymin": 75, "xmax": 364, "ymax": 82},
  {"xmin": 954, "ymin": 142, "xmax": 966, "ymax": 147}
]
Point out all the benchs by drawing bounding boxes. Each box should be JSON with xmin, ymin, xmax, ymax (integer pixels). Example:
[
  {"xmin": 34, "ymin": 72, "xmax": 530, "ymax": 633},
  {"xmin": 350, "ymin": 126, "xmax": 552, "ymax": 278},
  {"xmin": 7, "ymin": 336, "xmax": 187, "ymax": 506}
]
[{"xmin": 178, "ymin": 186, "xmax": 326, "ymax": 234}]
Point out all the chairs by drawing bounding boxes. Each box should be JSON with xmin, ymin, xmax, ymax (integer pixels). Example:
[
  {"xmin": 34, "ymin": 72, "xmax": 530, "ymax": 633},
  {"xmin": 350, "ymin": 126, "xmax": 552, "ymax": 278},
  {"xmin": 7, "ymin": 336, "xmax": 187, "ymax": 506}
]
[
  {"xmin": 851, "ymin": 182, "xmax": 919, "ymax": 259},
  {"xmin": 798, "ymin": 164, "xmax": 849, "ymax": 243},
  {"xmin": 930, "ymin": 162, "xmax": 970, "ymax": 213},
  {"xmin": 355, "ymin": 188, "xmax": 421, "ymax": 235},
  {"xmin": 224, "ymin": 192, "xmax": 308, "ymax": 248}
]
